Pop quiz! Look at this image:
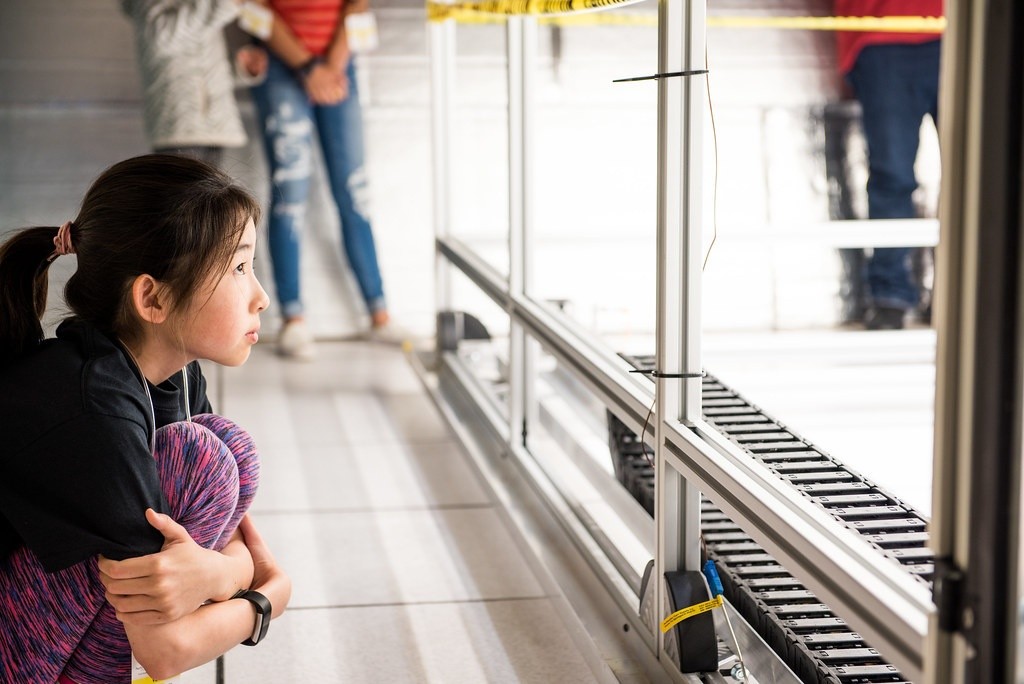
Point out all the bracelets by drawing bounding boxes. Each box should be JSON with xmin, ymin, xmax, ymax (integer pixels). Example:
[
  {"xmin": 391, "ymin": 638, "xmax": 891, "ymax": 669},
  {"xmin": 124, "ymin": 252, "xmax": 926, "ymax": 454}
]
[{"xmin": 293, "ymin": 57, "xmax": 318, "ymax": 78}]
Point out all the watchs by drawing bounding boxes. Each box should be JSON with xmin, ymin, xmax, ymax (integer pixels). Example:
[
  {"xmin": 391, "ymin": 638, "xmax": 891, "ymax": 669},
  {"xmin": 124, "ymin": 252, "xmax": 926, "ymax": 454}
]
[{"xmin": 231, "ymin": 589, "xmax": 272, "ymax": 646}]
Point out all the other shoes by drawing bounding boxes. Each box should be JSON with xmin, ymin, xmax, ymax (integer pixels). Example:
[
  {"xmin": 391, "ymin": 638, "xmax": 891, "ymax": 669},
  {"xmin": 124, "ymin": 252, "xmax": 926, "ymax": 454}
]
[
  {"xmin": 359, "ymin": 322, "xmax": 403, "ymax": 343},
  {"xmin": 281, "ymin": 321, "xmax": 313, "ymax": 352},
  {"xmin": 863, "ymin": 305, "xmax": 904, "ymax": 330}
]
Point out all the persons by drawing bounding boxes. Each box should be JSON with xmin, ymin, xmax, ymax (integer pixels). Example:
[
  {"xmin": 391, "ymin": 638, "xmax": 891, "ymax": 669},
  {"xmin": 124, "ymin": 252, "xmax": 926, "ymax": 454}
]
[
  {"xmin": 0, "ymin": 154, "xmax": 290, "ymax": 684},
  {"xmin": 122, "ymin": 0, "xmax": 249, "ymax": 168},
  {"xmin": 834, "ymin": 0, "xmax": 944, "ymax": 331},
  {"xmin": 252, "ymin": 0, "xmax": 419, "ymax": 362}
]
